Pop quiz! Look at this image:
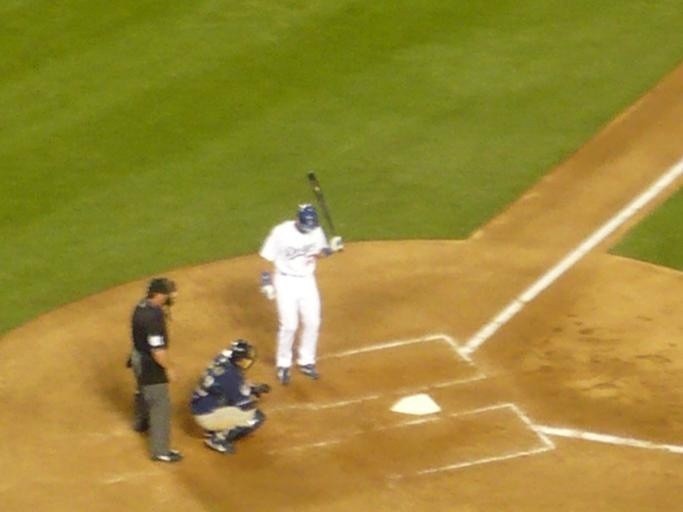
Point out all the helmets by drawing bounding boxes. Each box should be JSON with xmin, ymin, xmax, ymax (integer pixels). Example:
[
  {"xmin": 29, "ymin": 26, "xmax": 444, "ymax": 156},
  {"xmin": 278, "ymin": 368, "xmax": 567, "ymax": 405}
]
[
  {"xmin": 228, "ymin": 339, "xmax": 254, "ymax": 360},
  {"xmin": 295, "ymin": 205, "xmax": 320, "ymax": 232}
]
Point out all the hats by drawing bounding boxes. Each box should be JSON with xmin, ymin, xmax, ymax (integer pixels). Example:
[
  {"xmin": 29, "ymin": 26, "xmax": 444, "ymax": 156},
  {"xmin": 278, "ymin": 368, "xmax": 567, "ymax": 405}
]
[{"xmin": 147, "ymin": 276, "xmax": 178, "ymax": 295}]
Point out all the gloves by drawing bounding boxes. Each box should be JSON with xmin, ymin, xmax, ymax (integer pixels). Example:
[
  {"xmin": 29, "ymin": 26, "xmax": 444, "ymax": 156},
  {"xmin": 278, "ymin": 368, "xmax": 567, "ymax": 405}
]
[
  {"xmin": 322, "ymin": 236, "xmax": 344, "ymax": 257},
  {"xmin": 251, "ymin": 383, "xmax": 272, "ymax": 404},
  {"xmin": 259, "ymin": 272, "xmax": 275, "ymax": 300}
]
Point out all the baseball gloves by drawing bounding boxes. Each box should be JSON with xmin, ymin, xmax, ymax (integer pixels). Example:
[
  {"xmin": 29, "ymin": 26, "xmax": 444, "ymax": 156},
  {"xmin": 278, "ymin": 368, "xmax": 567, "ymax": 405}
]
[{"xmin": 250, "ymin": 385, "xmax": 270, "ymax": 397}]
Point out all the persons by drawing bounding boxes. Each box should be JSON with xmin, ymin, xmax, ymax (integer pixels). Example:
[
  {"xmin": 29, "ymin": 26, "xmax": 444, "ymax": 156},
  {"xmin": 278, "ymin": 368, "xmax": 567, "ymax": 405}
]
[
  {"xmin": 131, "ymin": 278, "xmax": 183, "ymax": 463},
  {"xmin": 190, "ymin": 340, "xmax": 271, "ymax": 454},
  {"xmin": 259, "ymin": 204, "xmax": 344, "ymax": 385}
]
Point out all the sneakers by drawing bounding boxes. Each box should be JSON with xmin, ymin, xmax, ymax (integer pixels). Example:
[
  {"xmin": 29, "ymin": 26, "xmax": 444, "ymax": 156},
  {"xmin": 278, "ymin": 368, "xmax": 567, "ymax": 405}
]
[
  {"xmin": 204, "ymin": 430, "xmax": 238, "ymax": 455},
  {"xmin": 275, "ymin": 363, "xmax": 321, "ymax": 385},
  {"xmin": 153, "ymin": 450, "xmax": 183, "ymax": 463}
]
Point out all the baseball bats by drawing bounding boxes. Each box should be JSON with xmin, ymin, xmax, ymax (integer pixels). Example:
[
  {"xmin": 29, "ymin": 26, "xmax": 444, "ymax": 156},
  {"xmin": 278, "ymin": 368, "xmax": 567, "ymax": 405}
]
[{"xmin": 307, "ymin": 172, "xmax": 344, "ymax": 251}]
